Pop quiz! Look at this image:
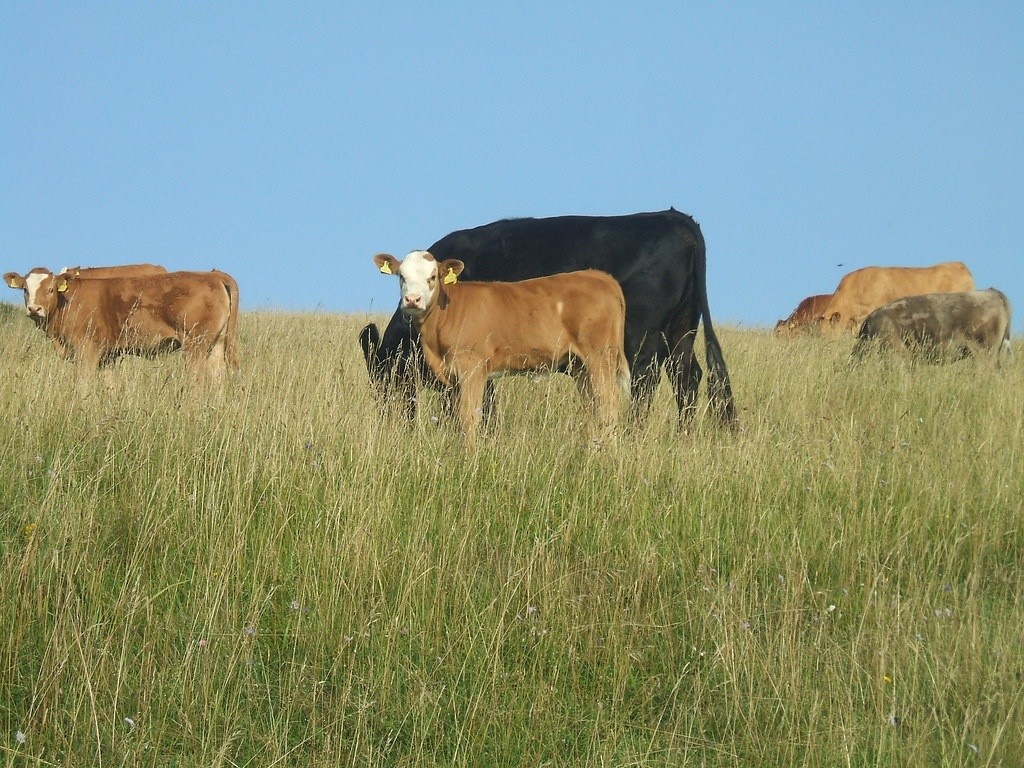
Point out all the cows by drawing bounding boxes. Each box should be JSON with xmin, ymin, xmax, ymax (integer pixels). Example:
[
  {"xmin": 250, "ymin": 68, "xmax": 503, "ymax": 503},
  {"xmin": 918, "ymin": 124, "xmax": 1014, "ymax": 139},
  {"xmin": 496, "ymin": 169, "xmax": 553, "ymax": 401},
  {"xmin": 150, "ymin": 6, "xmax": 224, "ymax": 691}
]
[
  {"xmin": 849, "ymin": 288, "xmax": 1015, "ymax": 373},
  {"xmin": 358, "ymin": 207, "xmax": 736, "ymax": 436},
  {"xmin": 2, "ymin": 264, "xmax": 240, "ymax": 388},
  {"xmin": 372, "ymin": 249, "xmax": 633, "ymax": 446},
  {"xmin": 773, "ymin": 263, "xmax": 975, "ymax": 341}
]
